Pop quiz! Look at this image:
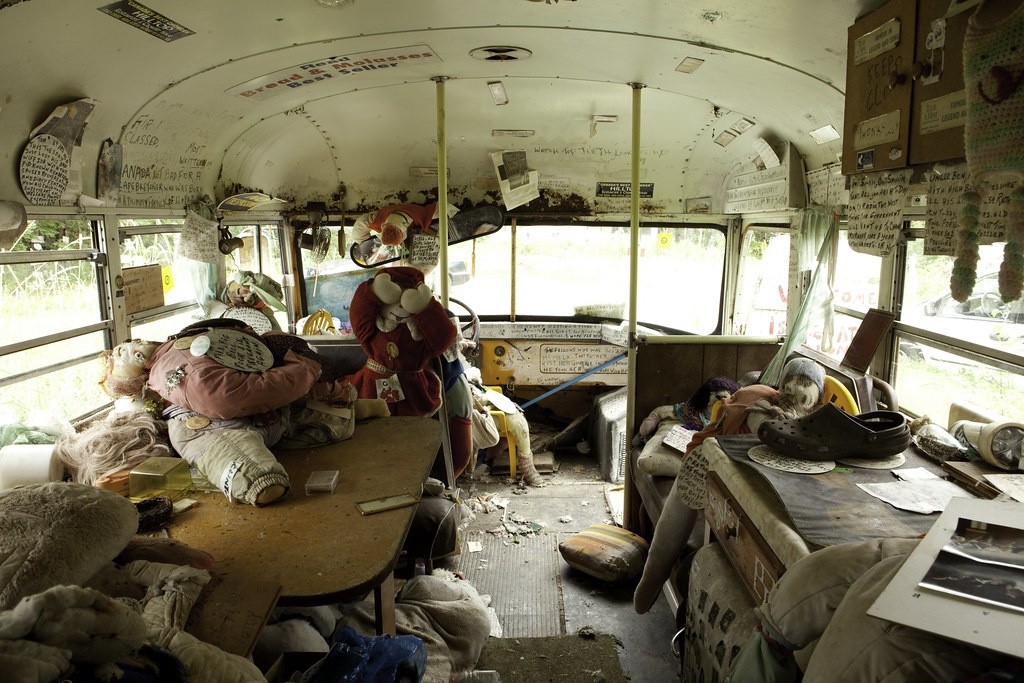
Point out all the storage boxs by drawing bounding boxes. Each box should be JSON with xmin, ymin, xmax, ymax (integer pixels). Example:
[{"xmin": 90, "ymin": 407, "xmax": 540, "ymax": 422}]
[{"xmin": 129, "ymin": 457, "xmax": 194, "ymax": 503}]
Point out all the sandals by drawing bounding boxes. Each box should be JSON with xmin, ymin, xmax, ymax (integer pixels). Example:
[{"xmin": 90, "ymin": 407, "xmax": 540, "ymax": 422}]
[{"xmin": 757, "ymin": 402, "xmax": 912, "ymax": 460}]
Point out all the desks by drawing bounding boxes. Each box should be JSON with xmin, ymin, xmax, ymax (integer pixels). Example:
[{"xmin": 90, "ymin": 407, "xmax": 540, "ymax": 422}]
[{"xmin": 137, "ymin": 414, "xmax": 444, "ymax": 660}]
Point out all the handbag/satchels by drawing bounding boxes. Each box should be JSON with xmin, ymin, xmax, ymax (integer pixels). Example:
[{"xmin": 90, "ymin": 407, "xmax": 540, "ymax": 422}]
[{"xmin": 472, "ymin": 396, "xmax": 500, "ymax": 449}]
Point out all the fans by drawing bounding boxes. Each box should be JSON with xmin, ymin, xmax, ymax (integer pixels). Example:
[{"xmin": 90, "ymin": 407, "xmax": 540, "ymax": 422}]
[{"xmin": 298, "ymin": 228, "xmax": 331, "ymax": 263}]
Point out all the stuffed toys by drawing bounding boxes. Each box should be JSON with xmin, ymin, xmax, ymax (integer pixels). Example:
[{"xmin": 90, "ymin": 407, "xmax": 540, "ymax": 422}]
[
  {"xmin": 352, "ymin": 196, "xmax": 459, "ymax": 244},
  {"xmin": 96, "ymin": 317, "xmax": 357, "ymax": 419},
  {"xmin": 342, "ymin": 267, "xmax": 457, "ymax": 420},
  {"xmin": 630, "ymin": 356, "xmax": 827, "ymax": 614}
]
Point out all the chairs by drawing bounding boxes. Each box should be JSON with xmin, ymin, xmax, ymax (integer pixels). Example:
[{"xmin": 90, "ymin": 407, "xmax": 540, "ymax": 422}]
[{"xmin": 487, "ymin": 386, "xmax": 517, "ymax": 480}]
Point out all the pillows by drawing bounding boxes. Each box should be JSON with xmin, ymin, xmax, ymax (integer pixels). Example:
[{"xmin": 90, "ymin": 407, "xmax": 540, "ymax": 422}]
[
  {"xmin": 637, "ymin": 420, "xmax": 699, "ymax": 476},
  {"xmin": 559, "ymin": 524, "xmax": 649, "ymax": 583}
]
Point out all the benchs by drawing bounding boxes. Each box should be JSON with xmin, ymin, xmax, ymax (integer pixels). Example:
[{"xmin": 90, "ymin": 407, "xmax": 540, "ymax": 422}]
[{"xmin": 635, "ymin": 347, "xmax": 900, "ymax": 560}]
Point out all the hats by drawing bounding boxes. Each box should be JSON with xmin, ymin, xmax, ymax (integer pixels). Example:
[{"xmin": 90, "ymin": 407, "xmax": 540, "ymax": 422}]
[{"xmin": 252, "ymin": 272, "xmax": 289, "ymax": 313}]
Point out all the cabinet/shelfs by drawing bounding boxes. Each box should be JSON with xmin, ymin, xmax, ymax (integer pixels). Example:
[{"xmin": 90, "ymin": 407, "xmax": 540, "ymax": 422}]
[
  {"xmin": 842, "ymin": 0, "xmax": 978, "ymax": 175},
  {"xmin": 703, "ymin": 436, "xmax": 977, "ymax": 606}
]
